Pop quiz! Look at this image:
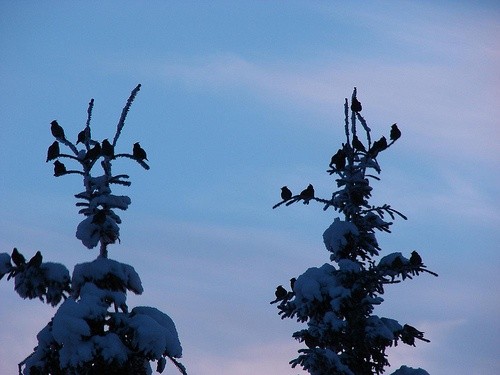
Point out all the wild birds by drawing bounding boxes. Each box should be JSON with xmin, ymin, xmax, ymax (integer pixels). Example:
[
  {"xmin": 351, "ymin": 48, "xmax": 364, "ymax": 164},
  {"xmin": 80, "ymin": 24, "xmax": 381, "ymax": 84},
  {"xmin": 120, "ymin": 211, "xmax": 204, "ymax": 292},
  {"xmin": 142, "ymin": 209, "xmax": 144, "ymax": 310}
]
[
  {"xmin": 11, "ymin": 248, "xmax": 43, "ymax": 269},
  {"xmin": 351, "ymin": 97, "xmax": 362, "ymax": 115},
  {"xmin": 275, "ymin": 278, "xmax": 296, "ymax": 298},
  {"xmin": 335, "ymin": 122, "xmax": 401, "ymax": 170},
  {"xmin": 280, "ymin": 184, "xmax": 314, "ymax": 202},
  {"xmin": 91, "ymin": 209, "xmax": 107, "ymax": 225},
  {"xmin": 392, "ymin": 251, "xmax": 422, "ymax": 271},
  {"xmin": 46, "ymin": 120, "xmax": 149, "ymax": 178}
]
